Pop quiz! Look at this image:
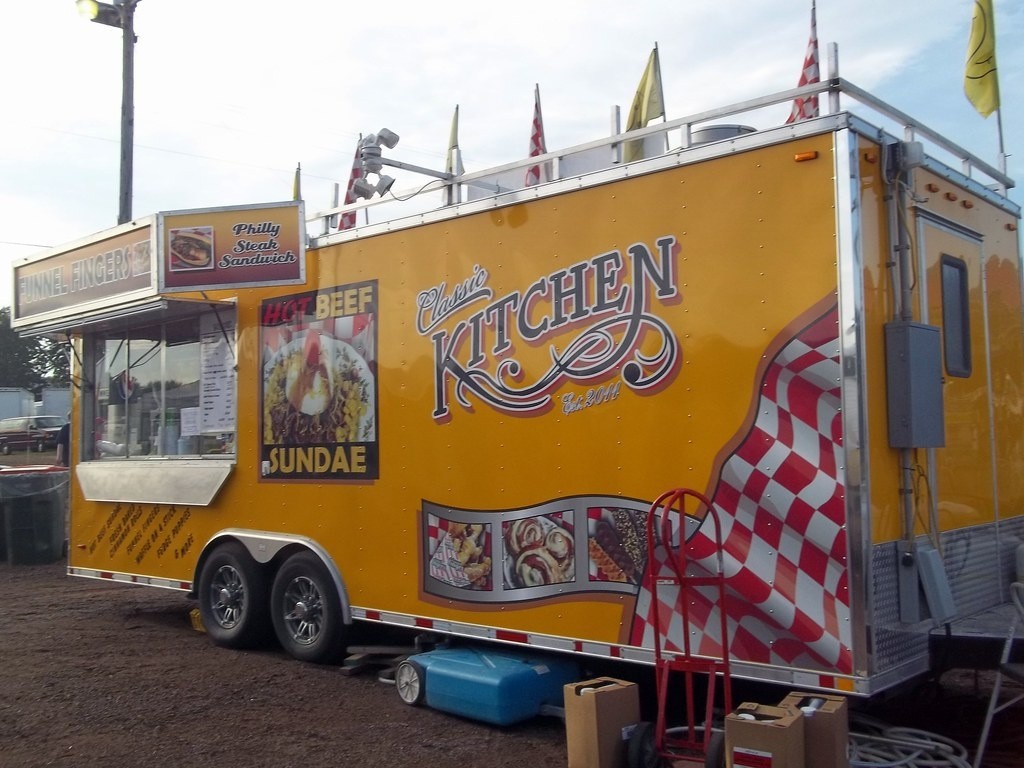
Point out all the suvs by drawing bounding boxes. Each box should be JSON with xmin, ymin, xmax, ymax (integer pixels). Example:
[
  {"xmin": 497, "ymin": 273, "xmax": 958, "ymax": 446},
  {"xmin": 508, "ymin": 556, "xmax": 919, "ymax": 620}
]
[{"xmin": 0, "ymin": 415, "xmax": 65, "ymax": 455}]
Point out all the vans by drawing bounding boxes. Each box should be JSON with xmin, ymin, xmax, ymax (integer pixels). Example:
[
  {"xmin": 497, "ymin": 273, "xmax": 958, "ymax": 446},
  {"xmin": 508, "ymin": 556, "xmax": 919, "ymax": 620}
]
[{"xmin": 66, "ymin": 38, "xmax": 1024, "ymax": 699}]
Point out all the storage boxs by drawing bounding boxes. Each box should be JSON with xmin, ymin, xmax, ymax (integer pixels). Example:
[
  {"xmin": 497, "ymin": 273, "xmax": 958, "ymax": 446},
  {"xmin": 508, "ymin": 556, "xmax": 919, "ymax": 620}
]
[
  {"xmin": 723, "ymin": 703, "xmax": 808, "ymax": 768},
  {"xmin": 778, "ymin": 690, "xmax": 850, "ymax": 766},
  {"xmin": 565, "ymin": 679, "xmax": 641, "ymax": 768}
]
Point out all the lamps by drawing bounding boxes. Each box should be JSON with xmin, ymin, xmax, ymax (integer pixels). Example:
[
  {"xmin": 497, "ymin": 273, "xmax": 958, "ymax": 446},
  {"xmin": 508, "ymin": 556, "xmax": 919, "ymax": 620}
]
[{"xmin": 349, "ymin": 128, "xmax": 402, "ymax": 199}]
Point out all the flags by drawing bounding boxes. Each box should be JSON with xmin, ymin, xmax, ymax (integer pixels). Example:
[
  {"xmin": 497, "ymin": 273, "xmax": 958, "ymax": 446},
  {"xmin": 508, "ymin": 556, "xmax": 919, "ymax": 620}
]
[
  {"xmin": 781, "ymin": 8, "xmax": 820, "ymax": 125},
  {"xmin": 620, "ymin": 48, "xmax": 665, "ymax": 164},
  {"xmin": 962, "ymin": 0, "xmax": 1001, "ymax": 120},
  {"xmin": 292, "ymin": 166, "xmax": 302, "ymax": 203},
  {"xmin": 524, "ymin": 87, "xmax": 547, "ymax": 187},
  {"xmin": 442, "ymin": 104, "xmax": 465, "ymax": 178},
  {"xmin": 338, "ymin": 142, "xmax": 366, "ymax": 230}
]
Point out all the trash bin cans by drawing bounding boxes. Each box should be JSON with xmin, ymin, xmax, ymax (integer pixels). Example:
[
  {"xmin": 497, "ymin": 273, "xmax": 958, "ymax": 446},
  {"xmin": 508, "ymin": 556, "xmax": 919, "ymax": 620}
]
[{"xmin": 0, "ymin": 471, "xmax": 68, "ymax": 566}]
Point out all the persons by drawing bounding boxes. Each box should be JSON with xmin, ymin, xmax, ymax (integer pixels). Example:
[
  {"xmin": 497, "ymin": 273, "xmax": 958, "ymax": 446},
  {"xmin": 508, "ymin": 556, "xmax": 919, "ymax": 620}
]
[{"xmin": 55, "ymin": 409, "xmax": 72, "ymax": 468}]
